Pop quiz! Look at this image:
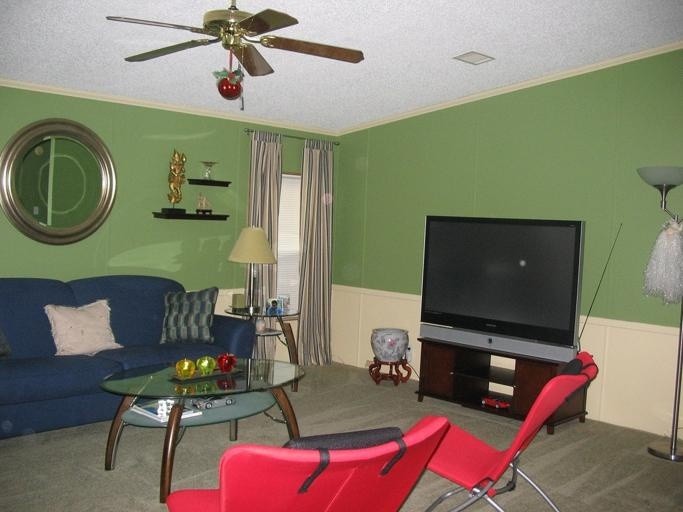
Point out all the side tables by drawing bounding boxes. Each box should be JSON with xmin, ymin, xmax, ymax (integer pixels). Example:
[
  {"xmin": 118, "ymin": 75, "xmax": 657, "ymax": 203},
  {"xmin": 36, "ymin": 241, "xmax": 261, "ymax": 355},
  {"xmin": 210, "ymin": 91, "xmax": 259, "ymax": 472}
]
[{"xmin": 224, "ymin": 305, "xmax": 307, "ymax": 393}]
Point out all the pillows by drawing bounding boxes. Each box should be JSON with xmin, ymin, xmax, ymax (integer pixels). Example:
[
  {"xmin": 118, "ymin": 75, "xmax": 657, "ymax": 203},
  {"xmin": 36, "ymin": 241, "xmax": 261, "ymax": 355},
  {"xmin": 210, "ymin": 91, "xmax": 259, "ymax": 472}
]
[
  {"xmin": 159, "ymin": 286, "xmax": 219, "ymax": 344},
  {"xmin": 43, "ymin": 300, "xmax": 124, "ymax": 356}
]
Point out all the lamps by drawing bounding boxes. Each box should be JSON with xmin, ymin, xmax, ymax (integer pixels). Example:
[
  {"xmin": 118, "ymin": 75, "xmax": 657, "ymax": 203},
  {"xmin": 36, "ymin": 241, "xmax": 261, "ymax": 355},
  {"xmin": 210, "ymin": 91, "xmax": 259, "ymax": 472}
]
[
  {"xmin": 636, "ymin": 165, "xmax": 683, "ymax": 210},
  {"xmin": 227, "ymin": 228, "xmax": 277, "ymax": 316}
]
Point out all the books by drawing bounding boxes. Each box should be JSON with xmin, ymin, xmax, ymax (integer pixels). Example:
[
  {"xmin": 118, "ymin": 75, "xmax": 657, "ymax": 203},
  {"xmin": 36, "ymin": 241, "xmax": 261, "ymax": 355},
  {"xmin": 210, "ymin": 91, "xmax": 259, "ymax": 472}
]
[{"xmin": 130, "ymin": 398, "xmax": 203, "ymax": 423}]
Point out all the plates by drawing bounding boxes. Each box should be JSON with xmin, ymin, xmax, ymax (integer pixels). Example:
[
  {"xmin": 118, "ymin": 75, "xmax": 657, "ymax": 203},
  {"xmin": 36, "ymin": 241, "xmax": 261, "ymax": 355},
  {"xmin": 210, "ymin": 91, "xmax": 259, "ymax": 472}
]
[{"xmin": 169, "ymin": 367, "xmax": 244, "ymax": 381}]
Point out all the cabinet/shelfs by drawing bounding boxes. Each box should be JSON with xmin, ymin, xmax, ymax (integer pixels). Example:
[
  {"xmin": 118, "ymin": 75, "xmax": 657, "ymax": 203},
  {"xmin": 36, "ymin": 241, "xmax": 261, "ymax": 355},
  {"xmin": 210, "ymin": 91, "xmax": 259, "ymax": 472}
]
[{"xmin": 414, "ymin": 337, "xmax": 589, "ymax": 434}]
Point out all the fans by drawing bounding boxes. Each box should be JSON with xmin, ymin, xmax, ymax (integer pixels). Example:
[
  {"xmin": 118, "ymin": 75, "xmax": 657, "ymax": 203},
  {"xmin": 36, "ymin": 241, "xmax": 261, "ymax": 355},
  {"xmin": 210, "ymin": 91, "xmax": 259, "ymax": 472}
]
[{"xmin": 106, "ymin": 7, "xmax": 364, "ymax": 78}]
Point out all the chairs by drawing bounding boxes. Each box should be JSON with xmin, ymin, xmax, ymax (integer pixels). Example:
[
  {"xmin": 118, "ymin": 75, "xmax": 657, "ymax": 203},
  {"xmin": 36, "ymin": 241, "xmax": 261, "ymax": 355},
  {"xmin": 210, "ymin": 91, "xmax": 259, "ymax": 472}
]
[
  {"xmin": 167, "ymin": 416, "xmax": 451, "ymax": 512},
  {"xmin": 404, "ymin": 351, "xmax": 598, "ymax": 512}
]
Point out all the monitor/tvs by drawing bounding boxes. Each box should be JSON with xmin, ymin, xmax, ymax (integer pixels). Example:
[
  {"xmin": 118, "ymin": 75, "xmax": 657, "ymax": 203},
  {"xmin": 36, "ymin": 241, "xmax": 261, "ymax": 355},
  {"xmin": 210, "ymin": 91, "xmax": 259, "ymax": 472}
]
[{"xmin": 418, "ymin": 214, "xmax": 586, "ymax": 365}]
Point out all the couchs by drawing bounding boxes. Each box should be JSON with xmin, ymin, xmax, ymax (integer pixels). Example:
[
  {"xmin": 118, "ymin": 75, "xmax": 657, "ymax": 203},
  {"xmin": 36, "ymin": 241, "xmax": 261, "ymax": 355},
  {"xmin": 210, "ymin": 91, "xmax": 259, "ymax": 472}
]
[{"xmin": 0, "ymin": 274, "xmax": 255, "ymax": 442}]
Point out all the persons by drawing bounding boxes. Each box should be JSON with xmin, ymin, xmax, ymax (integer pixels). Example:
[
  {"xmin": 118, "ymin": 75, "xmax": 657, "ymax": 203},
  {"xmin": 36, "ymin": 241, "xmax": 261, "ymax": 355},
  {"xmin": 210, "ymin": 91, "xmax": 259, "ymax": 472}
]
[{"xmin": 268, "ymin": 301, "xmax": 282, "ymax": 315}]
